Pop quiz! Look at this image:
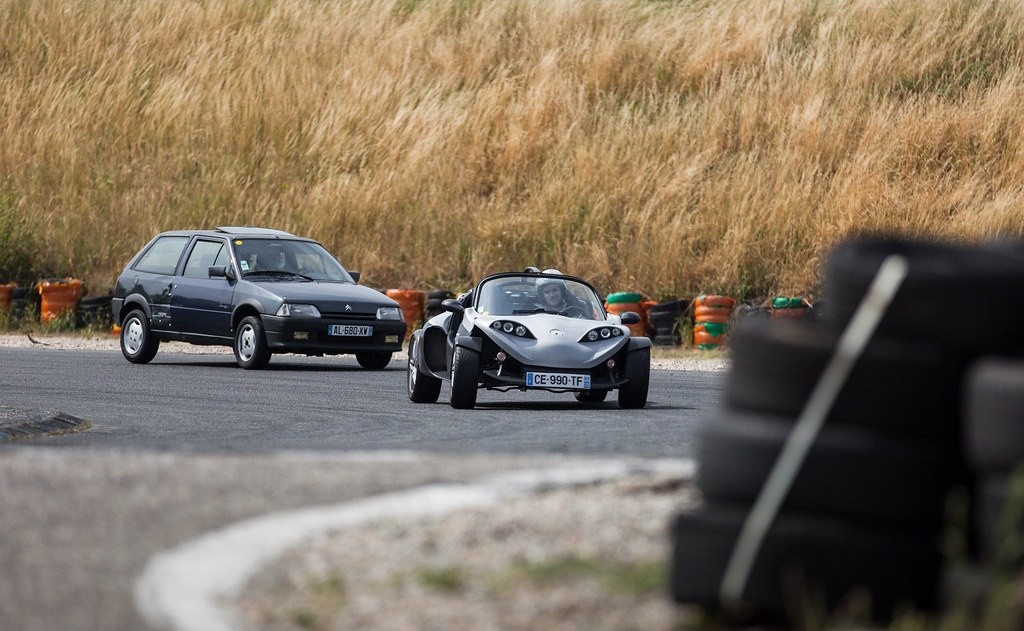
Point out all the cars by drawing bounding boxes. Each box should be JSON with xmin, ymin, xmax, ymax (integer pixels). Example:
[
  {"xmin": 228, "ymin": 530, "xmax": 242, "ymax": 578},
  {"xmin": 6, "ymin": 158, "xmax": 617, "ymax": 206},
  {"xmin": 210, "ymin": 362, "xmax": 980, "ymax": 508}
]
[{"xmin": 111, "ymin": 227, "xmax": 406, "ymax": 370}]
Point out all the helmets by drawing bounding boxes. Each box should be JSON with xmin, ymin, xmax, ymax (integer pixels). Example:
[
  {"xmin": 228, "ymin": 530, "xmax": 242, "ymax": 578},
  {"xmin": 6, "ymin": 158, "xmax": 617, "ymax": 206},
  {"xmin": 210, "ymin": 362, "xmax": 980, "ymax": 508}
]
[{"xmin": 535, "ymin": 270, "xmax": 566, "ymax": 296}]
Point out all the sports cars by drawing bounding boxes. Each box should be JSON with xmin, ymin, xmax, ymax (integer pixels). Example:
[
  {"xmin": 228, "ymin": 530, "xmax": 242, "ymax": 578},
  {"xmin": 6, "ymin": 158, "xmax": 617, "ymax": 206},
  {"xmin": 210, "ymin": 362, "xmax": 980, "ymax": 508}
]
[{"xmin": 408, "ymin": 266, "xmax": 652, "ymax": 408}]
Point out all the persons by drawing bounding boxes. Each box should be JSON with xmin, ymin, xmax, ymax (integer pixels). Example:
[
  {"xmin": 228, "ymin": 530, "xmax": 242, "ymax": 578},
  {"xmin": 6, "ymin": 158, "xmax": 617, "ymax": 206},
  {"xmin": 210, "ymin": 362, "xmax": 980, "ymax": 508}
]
[
  {"xmin": 536, "ymin": 268, "xmax": 586, "ymax": 320},
  {"xmin": 269, "ymin": 250, "xmax": 286, "ymax": 272}
]
